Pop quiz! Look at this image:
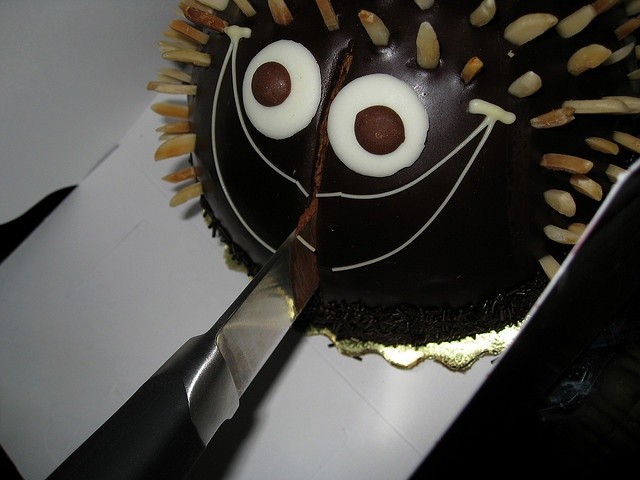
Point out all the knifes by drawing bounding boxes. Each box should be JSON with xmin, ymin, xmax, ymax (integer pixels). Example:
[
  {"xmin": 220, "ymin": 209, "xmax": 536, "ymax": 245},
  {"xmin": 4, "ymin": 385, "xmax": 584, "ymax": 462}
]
[{"xmin": 48, "ymin": 212, "xmax": 325, "ymax": 480}]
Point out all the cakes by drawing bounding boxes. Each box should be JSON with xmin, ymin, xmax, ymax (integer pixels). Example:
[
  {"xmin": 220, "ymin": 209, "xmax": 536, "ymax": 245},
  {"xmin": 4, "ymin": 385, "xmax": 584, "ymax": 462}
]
[{"xmin": 148, "ymin": 0, "xmax": 639, "ymax": 373}]
